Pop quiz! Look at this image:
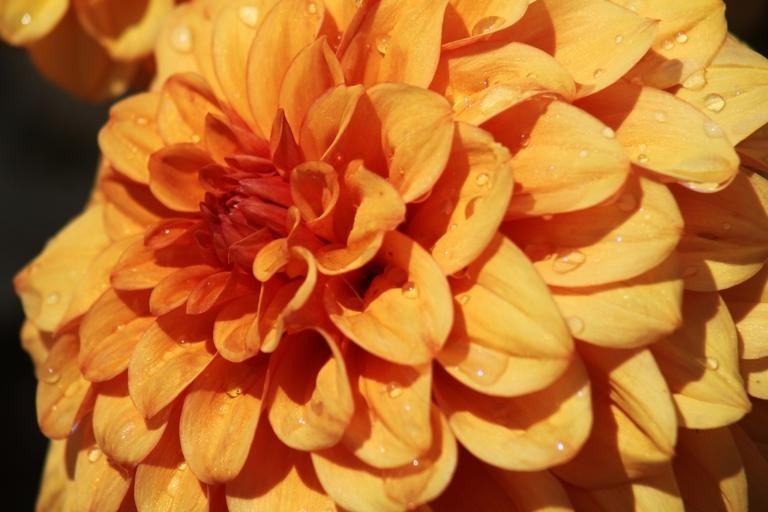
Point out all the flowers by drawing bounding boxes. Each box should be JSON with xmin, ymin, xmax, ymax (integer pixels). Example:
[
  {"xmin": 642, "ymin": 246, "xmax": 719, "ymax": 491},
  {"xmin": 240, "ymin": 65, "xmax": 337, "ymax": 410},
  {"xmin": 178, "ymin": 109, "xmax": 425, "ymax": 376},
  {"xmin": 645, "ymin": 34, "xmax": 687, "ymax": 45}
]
[{"xmin": 1, "ymin": 0, "xmax": 767, "ymax": 512}]
